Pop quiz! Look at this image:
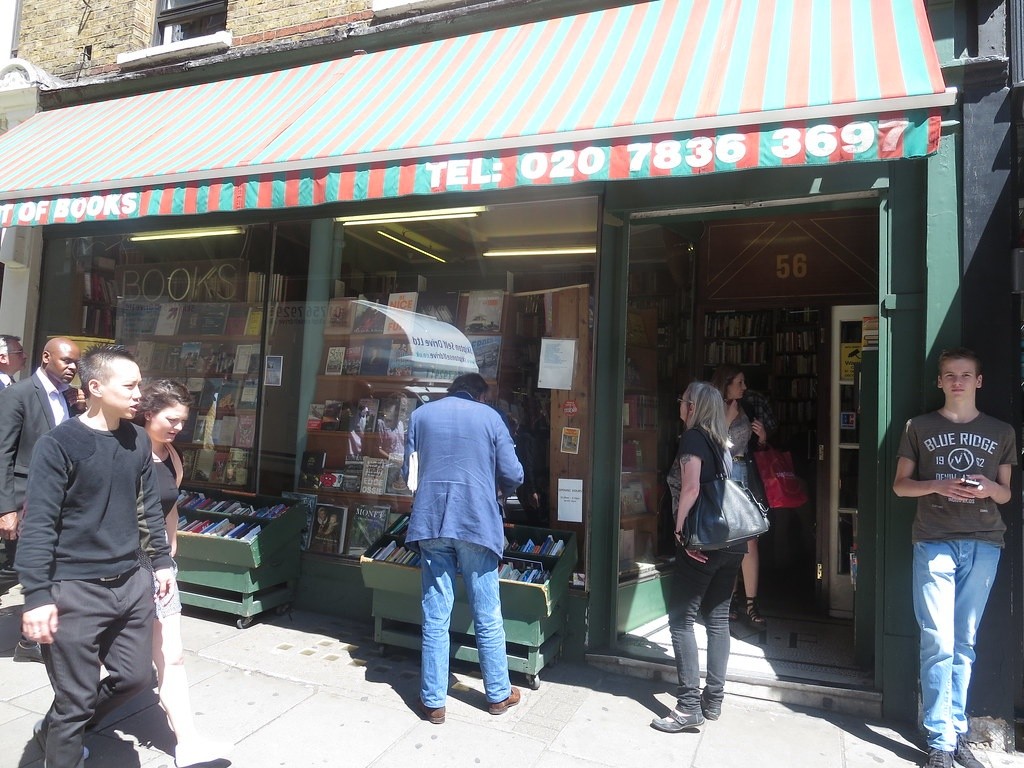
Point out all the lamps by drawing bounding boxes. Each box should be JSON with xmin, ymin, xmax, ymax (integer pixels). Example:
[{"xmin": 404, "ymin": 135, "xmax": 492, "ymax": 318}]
[
  {"xmin": 126, "ymin": 229, "xmax": 247, "ymax": 242},
  {"xmin": 333, "ymin": 207, "xmax": 490, "ymax": 227},
  {"xmin": 483, "ymin": 245, "xmax": 598, "ymax": 257}
]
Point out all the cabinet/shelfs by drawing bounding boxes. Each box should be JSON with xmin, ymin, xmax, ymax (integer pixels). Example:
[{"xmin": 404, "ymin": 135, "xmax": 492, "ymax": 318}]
[
  {"xmin": 296, "ymin": 293, "xmax": 507, "ymax": 502},
  {"xmin": 113, "ymin": 227, "xmax": 276, "ymax": 492},
  {"xmin": 774, "ymin": 305, "xmax": 822, "ymax": 464},
  {"xmin": 586, "ymin": 287, "xmax": 658, "ymax": 565},
  {"xmin": 693, "ymin": 303, "xmax": 772, "ymax": 428},
  {"xmin": 174, "ymin": 483, "xmax": 305, "ymax": 628},
  {"xmin": 830, "ymin": 304, "xmax": 879, "ymax": 621},
  {"xmin": 361, "ymin": 513, "xmax": 577, "ymax": 690}
]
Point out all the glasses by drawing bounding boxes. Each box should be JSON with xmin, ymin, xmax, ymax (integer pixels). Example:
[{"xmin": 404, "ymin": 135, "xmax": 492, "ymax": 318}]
[{"xmin": 4, "ymin": 348, "xmax": 24, "ymax": 355}]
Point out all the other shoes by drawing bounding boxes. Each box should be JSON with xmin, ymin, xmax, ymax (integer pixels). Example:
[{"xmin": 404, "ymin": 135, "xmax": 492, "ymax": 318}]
[
  {"xmin": 651, "ymin": 709, "xmax": 705, "ymax": 732},
  {"xmin": 13, "ymin": 642, "xmax": 45, "ymax": 664},
  {"xmin": 175, "ymin": 741, "xmax": 233, "ymax": 768},
  {"xmin": 700, "ymin": 694, "xmax": 722, "ymax": 720}
]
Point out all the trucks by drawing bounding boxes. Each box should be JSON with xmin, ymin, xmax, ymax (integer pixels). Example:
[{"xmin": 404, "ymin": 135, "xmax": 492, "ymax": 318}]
[{"xmin": 125, "ymin": 297, "xmax": 481, "ymax": 383}]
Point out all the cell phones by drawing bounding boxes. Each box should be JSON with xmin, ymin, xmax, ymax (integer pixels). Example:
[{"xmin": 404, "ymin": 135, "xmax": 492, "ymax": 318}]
[{"xmin": 961, "ymin": 480, "xmax": 980, "ymax": 488}]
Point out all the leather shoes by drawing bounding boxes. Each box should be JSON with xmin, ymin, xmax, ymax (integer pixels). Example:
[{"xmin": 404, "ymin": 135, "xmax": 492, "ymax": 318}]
[
  {"xmin": 489, "ymin": 686, "xmax": 520, "ymax": 715},
  {"xmin": 416, "ymin": 694, "xmax": 446, "ymax": 724}
]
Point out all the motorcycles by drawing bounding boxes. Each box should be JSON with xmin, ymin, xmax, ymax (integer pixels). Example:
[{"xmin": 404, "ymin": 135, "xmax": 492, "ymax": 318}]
[{"xmin": 310, "ymin": 365, "xmax": 453, "ymax": 493}]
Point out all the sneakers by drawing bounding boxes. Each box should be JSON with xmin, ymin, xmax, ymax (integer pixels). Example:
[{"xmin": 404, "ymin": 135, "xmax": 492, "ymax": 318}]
[
  {"xmin": 952, "ymin": 734, "xmax": 983, "ymax": 768},
  {"xmin": 922, "ymin": 746, "xmax": 954, "ymax": 768}
]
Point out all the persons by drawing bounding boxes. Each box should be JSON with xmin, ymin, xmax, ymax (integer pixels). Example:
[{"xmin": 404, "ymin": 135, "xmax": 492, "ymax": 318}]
[
  {"xmin": 402, "ymin": 373, "xmax": 525, "ymax": 724},
  {"xmin": 652, "ymin": 383, "xmax": 749, "ymax": 733},
  {"xmin": 711, "ymin": 362, "xmax": 781, "ymax": 627},
  {"xmin": 893, "ymin": 344, "xmax": 1018, "ymax": 768},
  {"xmin": 0, "ymin": 335, "xmax": 233, "ymax": 768},
  {"xmin": 372, "ymin": 390, "xmax": 407, "ymax": 462}
]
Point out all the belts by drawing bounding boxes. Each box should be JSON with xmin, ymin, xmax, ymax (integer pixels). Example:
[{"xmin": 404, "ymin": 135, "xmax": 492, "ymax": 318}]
[{"xmin": 97, "ymin": 575, "xmax": 124, "ymax": 582}]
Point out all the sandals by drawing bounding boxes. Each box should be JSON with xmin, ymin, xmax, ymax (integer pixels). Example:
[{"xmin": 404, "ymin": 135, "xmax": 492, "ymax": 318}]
[
  {"xmin": 745, "ymin": 596, "xmax": 767, "ymax": 628},
  {"xmin": 728, "ymin": 592, "xmax": 740, "ymax": 621}
]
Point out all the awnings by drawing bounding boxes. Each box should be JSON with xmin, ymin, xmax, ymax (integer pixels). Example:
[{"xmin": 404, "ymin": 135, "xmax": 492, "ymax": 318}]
[{"xmin": 0, "ymin": 0, "xmax": 956, "ymax": 226}]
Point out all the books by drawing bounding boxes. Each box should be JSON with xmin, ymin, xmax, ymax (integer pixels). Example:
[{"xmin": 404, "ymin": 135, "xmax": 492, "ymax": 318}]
[
  {"xmin": 278, "ymin": 489, "xmax": 400, "ymax": 561},
  {"xmin": 625, "ymin": 261, "xmax": 691, "ymax": 398},
  {"xmin": 80, "ymin": 248, "xmax": 144, "ymax": 339},
  {"xmin": 700, "ymin": 311, "xmax": 828, "ymax": 459},
  {"xmin": 175, "ymin": 488, "xmax": 289, "ymax": 520},
  {"xmin": 108, "ymin": 267, "xmax": 288, "ymax": 486},
  {"xmin": 371, "ymin": 514, "xmax": 565, "ymax": 585},
  {"xmin": 300, "ymin": 289, "xmax": 507, "ymax": 494},
  {"xmin": 177, "ymin": 516, "xmax": 261, "ymax": 541}
]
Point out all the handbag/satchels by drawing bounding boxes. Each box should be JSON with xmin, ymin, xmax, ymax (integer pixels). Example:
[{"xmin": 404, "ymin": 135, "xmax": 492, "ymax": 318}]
[
  {"xmin": 679, "ymin": 426, "xmax": 771, "ymax": 551},
  {"xmin": 753, "ymin": 441, "xmax": 809, "ymax": 509}
]
